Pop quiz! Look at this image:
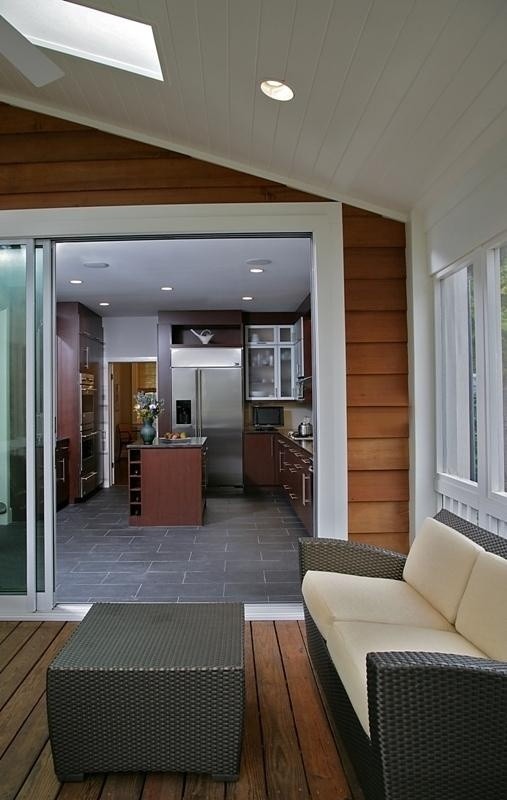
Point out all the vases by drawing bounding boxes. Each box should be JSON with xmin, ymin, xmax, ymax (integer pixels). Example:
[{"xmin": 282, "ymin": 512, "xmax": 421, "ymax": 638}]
[{"xmin": 138, "ymin": 418, "xmax": 156, "ymax": 445}]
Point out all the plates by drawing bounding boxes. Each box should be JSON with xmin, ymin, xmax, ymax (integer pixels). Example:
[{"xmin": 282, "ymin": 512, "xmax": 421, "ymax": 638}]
[{"xmin": 158, "ymin": 436, "xmax": 191, "ymax": 442}]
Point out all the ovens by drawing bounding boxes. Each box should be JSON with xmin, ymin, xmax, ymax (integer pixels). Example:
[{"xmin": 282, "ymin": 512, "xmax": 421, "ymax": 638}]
[{"xmin": 80, "ymin": 371, "xmax": 99, "ymax": 498}]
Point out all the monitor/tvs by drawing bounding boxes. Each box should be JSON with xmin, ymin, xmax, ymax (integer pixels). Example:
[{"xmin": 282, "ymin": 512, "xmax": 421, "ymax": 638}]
[{"xmin": 253, "ymin": 406, "xmax": 284, "ymax": 431}]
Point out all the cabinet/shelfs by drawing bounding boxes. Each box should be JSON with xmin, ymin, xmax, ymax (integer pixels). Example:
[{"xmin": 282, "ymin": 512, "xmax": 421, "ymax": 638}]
[
  {"xmin": 126, "ymin": 435, "xmax": 207, "ymax": 527},
  {"xmin": 275, "ymin": 434, "xmax": 314, "ymax": 539},
  {"xmin": 23, "ymin": 433, "xmax": 70, "ymax": 519},
  {"xmin": 55, "ymin": 303, "xmax": 106, "ymax": 505},
  {"xmin": 245, "ymin": 316, "xmax": 312, "ymax": 403},
  {"xmin": 155, "ymin": 310, "xmax": 242, "ymax": 347},
  {"xmin": 242, "ymin": 433, "xmax": 275, "ymax": 488}
]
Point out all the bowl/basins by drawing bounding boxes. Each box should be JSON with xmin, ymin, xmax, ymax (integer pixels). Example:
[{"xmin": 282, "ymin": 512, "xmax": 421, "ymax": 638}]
[{"xmin": 249, "ymin": 391, "xmax": 265, "ymax": 397}]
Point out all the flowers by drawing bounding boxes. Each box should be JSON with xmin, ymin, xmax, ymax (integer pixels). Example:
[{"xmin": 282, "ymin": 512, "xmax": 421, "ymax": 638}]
[{"xmin": 131, "ymin": 390, "xmax": 167, "ymax": 423}]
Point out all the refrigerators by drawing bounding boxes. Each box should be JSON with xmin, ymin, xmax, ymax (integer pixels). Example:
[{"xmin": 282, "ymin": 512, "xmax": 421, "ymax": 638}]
[{"xmin": 170, "ymin": 346, "xmax": 244, "ymax": 497}]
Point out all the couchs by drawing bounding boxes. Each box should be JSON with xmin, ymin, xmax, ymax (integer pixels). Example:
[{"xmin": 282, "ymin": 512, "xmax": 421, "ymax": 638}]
[{"xmin": 295, "ymin": 506, "xmax": 506, "ymax": 799}]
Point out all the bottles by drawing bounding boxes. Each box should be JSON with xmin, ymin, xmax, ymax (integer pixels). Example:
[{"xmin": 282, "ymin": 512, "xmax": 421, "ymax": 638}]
[
  {"xmin": 251, "ymin": 350, "xmax": 274, "ymax": 368},
  {"xmin": 250, "ymin": 334, "xmax": 259, "ymax": 342}
]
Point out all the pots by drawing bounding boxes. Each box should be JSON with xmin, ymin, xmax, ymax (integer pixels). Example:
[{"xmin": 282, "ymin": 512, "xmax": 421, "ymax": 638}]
[{"xmin": 297, "ymin": 420, "xmax": 313, "ymax": 437}]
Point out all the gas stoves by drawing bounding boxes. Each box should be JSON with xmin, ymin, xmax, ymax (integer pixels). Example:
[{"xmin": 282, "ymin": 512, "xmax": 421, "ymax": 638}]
[{"xmin": 287, "ymin": 431, "xmax": 313, "ymax": 441}]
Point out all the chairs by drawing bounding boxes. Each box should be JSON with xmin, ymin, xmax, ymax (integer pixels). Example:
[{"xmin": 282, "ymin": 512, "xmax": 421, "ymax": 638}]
[{"xmin": 117, "ymin": 423, "xmax": 141, "ymax": 461}]
